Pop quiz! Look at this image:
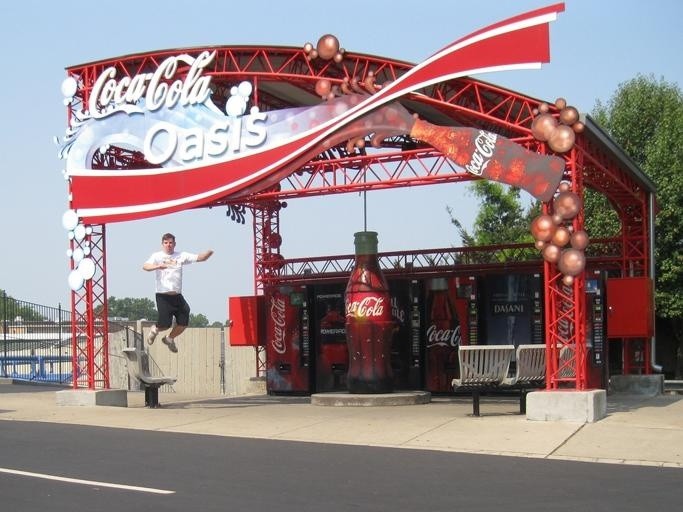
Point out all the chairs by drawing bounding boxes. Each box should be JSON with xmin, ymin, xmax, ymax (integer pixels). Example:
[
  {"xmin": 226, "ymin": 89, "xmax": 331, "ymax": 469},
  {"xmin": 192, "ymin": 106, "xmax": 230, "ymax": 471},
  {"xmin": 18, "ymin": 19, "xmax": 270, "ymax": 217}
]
[
  {"xmin": 449, "ymin": 343, "xmax": 594, "ymax": 418},
  {"xmin": 123, "ymin": 346, "xmax": 178, "ymax": 410}
]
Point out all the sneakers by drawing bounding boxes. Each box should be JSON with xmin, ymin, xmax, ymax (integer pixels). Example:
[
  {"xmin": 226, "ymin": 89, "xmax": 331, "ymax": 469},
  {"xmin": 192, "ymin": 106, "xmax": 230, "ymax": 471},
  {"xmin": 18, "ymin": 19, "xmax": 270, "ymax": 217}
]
[
  {"xmin": 146, "ymin": 324, "xmax": 159, "ymax": 346},
  {"xmin": 161, "ymin": 334, "xmax": 178, "ymax": 353}
]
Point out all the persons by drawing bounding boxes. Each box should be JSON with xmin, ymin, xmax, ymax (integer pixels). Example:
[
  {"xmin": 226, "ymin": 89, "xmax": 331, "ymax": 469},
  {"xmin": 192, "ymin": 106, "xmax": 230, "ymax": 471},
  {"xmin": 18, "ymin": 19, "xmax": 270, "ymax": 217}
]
[{"xmin": 144, "ymin": 232, "xmax": 214, "ymax": 354}]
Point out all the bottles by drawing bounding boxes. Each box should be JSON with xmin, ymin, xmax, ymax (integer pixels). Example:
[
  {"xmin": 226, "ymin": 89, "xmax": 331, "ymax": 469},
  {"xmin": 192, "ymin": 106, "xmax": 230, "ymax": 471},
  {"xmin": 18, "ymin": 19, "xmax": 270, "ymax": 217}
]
[
  {"xmin": 346, "ymin": 231, "xmax": 393, "ymax": 391},
  {"xmin": 407, "ymin": 119, "xmax": 566, "ymax": 202},
  {"xmin": 425, "ymin": 280, "xmax": 463, "ymax": 391}
]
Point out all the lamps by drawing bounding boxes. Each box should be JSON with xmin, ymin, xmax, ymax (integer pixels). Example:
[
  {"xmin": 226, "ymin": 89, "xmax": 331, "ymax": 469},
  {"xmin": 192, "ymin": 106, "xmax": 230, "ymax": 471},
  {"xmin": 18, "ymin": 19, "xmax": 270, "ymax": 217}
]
[{"xmin": 220, "ymin": 203, "xmax": 250, "ymax": 225}]
